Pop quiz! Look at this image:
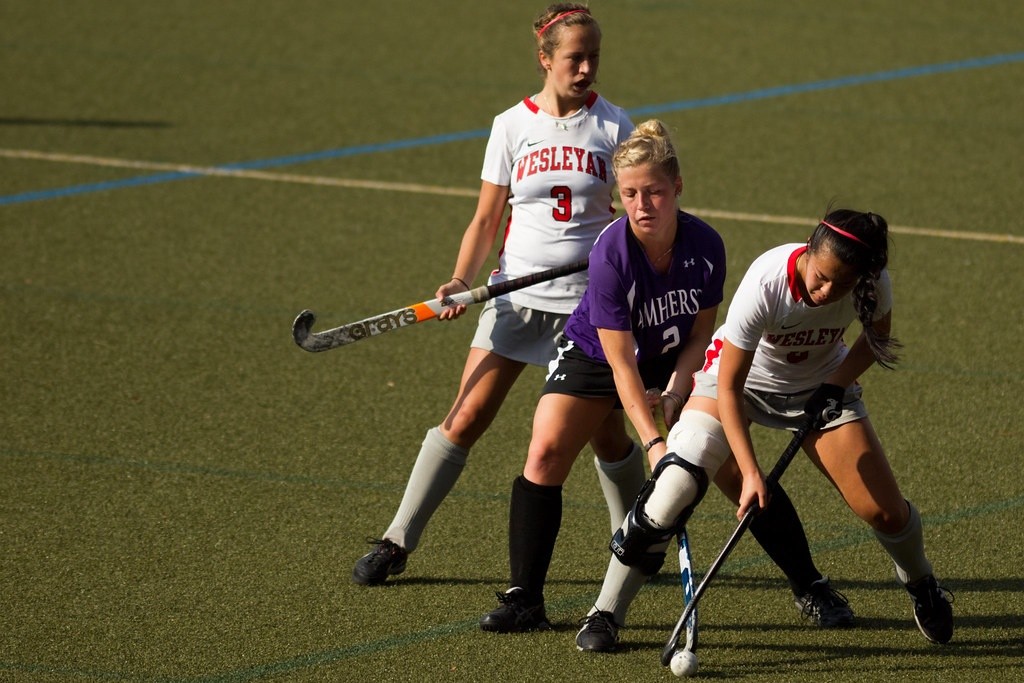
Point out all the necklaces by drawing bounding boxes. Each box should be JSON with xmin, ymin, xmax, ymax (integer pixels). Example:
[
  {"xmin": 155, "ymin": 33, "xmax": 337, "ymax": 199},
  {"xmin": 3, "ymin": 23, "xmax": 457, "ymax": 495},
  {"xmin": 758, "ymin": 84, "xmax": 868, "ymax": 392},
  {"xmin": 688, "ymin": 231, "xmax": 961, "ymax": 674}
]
[
  {"xmin": 541, "ymin": 91, "xmax": 574, "ymax": 130},
  {"xmin": 797, "ymin": 252, "xmax": 806, "ymax": 270},
  {"xmin": 653, "ymin": 247, "xmax": 671, "ymax": 267}
]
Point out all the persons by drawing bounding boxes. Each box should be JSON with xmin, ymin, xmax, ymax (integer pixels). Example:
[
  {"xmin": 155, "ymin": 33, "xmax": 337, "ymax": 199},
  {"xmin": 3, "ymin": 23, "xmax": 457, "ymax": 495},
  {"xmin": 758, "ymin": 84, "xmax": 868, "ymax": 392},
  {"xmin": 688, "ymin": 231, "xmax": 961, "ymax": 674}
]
[
  {"xmin": 481, "ymin": 118, "xmax": 859, "ymax": 634},
  {"xmin": 572, "ymin": 210, "xmax": 954, "ymax": 653},
  {"xmin": 351, "ymin": 4, "xmax": 645, "ymax": 585}
]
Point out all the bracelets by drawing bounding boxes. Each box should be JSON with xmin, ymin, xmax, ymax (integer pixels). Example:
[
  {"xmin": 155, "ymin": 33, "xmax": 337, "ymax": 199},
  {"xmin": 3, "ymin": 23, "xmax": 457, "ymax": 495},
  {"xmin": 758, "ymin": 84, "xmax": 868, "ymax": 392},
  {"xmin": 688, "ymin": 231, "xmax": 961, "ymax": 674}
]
[
  {"xmin": 660, "ymin": 390, "xmax": 685, "ymax": 408},
  {"xmin": 644, "ymin": 436, "xmax": 665, "ymax": 453},
  {"xmin": 450, "ymin": 277, "xmax": 471, "ymax": 291}
]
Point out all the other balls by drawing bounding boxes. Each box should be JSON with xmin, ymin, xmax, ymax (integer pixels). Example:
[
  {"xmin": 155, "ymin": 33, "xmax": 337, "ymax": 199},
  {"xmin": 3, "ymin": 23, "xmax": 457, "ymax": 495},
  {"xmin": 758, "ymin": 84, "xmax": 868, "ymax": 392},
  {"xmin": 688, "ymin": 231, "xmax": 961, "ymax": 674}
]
[{"xmin": 670, "ymin": 650, "xmax": 699, "ymax": 678}]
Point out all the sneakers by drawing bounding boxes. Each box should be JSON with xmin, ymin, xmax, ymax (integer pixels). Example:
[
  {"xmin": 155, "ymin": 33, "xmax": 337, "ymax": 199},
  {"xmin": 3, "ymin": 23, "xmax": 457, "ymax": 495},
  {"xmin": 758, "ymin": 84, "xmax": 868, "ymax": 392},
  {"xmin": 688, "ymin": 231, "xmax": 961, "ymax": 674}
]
[
  {"xmin": 575, "ymin": 603, "xmax": 622, "ymax": 653},
  {"xmin": 479, "ymin": 586, "xmax": 548, "ymax": 632},
  {"xmin": 896, "ymin": 570, "xmax": 958, "ymax": 644},
  {"xmin": 789, "ymin": 577, "xmax": 859, "ymax": 630},
  {"xmin": 350, "ymin": 536, "xmax": 409, "ymax": 587}
]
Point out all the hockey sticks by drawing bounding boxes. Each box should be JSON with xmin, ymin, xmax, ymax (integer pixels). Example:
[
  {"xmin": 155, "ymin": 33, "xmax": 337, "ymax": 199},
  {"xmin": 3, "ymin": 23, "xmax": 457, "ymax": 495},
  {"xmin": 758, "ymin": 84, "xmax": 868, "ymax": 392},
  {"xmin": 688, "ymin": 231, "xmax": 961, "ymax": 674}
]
[
  {"xmin": 642, "ymin": 385, "xmax": 700, "ymax": 653},
  {"xmin": 288, "ymin": 258, "xmax": 592, "ymax": 354},
  {"xmin": 657, "ymin": 410, "xmax": 817, "ymax": 668}
]
